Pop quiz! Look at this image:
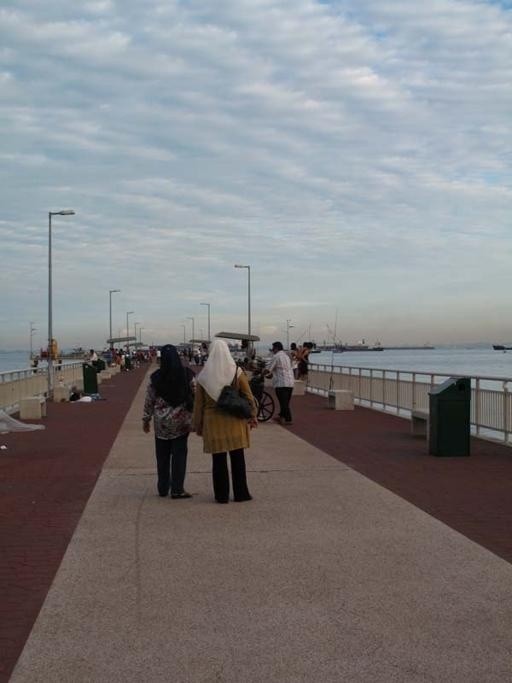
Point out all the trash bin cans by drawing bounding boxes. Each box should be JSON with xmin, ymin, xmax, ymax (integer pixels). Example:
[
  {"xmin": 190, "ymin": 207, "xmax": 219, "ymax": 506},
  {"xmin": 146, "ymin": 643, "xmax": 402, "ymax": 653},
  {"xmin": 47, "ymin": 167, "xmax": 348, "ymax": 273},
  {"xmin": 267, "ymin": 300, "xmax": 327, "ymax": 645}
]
[
  {"xmin": 426, "ymin": 375, "xmax": 473, "ymax": 459},
  {"xmin": 125, "ymin": 356, "xmax": 132, "ymax": 368},
  {"xmin": 81, "ymin": 361, "xmax": 98, "ymax": 396}
]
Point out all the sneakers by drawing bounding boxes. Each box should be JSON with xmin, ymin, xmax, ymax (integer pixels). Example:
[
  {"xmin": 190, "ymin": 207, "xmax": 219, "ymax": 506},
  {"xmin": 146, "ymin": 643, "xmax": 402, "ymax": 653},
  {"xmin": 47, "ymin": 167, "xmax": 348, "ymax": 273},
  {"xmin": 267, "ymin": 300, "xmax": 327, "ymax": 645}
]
[
  {"xmin": 280, "ymin": 420, "xmax": 294, "ymax": 426},
  {"xmin": 271, "ymin": 416, "xmax": 283, "ymax": 425},
  {"xmin": 171, "ymin": 492, "xmax": 194, "ymax": 499}
]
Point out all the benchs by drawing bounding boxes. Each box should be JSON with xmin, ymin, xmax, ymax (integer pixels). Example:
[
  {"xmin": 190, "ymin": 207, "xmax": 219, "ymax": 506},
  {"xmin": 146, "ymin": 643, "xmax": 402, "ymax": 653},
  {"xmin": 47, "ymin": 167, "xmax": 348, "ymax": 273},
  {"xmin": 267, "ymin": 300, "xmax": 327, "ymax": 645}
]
[
  {"xmin": 20, "ymin": 394, "xmax": 47, "ymax": 420},
  {"xmin": 328, "ymin": 389, "xmax": 354, "ymax": 411},
  {"xmin": 411, "ymin": 407, "xmax": 429, "ymax": 440},
  {"xmin": 292, "ymin": 378, "xmax": 305, "ymax": 396},
  {"xmin": 53, "ymin": 363, "xmax": 121, "ymax": 403}
]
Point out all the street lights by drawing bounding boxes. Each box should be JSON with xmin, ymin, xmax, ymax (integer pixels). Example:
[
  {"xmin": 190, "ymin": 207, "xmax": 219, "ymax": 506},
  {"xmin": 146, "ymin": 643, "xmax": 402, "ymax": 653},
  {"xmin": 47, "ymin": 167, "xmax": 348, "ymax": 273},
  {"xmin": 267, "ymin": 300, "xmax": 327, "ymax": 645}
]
[
  {"xmin": 108, "ymin": 288, "xmax": 121, "ymax": 351},
  {"xmin": 139, "ymin": 326, "xmax": 147, "ymax": 344},
  {"xmin": 234, "ymin": 262, "xmax": 252, "ymax": 338},
  {"xmin": 180, "ymin": 323, "xmax": 186, "ymax": 342},
  {"xmin": 200, "ymin": 302, "xmax": 210, "ymax": 341},
  {"xmin": 135, "ymin": 321, "xmax": 141, "ymax": 346},
  {"xmin": 187, "ymin": 316, "xmax": 195, "ymax": 344},
  {"xmin": 48, "ymin": 209, "xmax": 75, "ymax": 395},
  {"xmin": 31, "ymin": 321, "xmax": 39, "ymax": 361},
  {"xmin": 126, "ymin": 311, "xmax": 134, "ymax": 349}
]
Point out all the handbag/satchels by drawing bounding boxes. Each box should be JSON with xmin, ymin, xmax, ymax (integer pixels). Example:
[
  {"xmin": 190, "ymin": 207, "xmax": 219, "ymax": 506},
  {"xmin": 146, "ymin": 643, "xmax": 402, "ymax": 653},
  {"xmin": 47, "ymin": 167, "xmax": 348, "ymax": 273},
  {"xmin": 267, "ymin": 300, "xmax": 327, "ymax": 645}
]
[{"xmin": 215, "ymin": 384, "xmax": 252, "ymax": 421}]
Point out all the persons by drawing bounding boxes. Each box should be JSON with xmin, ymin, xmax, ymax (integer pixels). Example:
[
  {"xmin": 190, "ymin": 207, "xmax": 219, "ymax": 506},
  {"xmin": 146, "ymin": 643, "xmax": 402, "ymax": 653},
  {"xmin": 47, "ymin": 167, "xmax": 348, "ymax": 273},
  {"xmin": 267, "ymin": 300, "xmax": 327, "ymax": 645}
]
[
  {"xmin": 191, "ymin": 339, "xmax": 259, "ymax": 505},
  {"xmin": 290, "ymin": 339, "xmax": 317, "ymax": 379},
  {"xmin": 85, "ymin": 343, "xmax": 209, "ymax": 369},
  {"xmin": 142, "ymin": 345, "xmax": 198, "ymax": 499},
  {"xmin": 266, "ymin": 341, "xmax": 297, "ymax": 426}
]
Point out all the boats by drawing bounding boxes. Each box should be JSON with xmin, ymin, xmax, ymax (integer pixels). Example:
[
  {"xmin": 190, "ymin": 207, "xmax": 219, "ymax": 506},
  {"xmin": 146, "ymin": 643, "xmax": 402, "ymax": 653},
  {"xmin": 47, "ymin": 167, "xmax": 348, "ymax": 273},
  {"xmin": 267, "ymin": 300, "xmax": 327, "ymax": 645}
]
[
  {"xmin": 493, "ymin": 344, "xmax": 511, "ymax": 351},
  {"xmin": 310, "ymin": 308, "xmax": 386, "ymax": 353},
  {"xmin": 34, "ymin": 349, "xmax": 117, "ymax": 360}
]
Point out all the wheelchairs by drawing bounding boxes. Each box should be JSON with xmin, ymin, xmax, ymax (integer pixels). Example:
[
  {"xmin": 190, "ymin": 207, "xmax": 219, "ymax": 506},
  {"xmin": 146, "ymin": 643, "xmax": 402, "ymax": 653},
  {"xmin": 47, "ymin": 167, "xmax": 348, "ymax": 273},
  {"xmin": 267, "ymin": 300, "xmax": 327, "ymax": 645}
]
[{"xmin": 250, "ymin": 371, "xmax": 275, "ymax": 422}]
